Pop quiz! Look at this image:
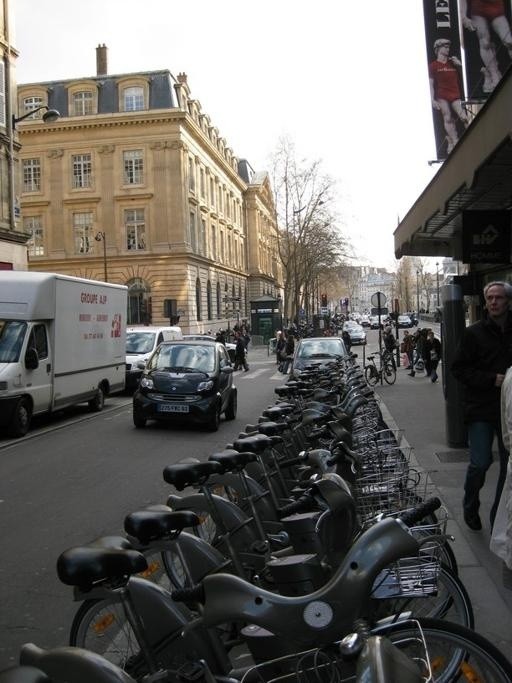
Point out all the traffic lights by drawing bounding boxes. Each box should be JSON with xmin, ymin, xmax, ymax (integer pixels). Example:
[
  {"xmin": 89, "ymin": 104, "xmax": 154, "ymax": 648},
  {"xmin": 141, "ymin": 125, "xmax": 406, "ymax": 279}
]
[{"xmin": 322, "ymin": 294, "xmax": 327, "ymax": 305}]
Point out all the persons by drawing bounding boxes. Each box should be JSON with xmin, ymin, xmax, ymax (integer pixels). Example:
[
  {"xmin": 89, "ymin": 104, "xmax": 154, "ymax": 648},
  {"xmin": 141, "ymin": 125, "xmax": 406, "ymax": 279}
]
[
  {"xmin": 428, "ymin": 38, "xmax": 472, "ymax": 148},
  {"xmin": 459, "ymin": 0, "xmax": 512, "ymax": 87},
  {"xmin": 383, "ymin": 308, "xmax": 442, "ymax": 383},
  {"xmin": 488, "ymin": 368, "xmax": 512, "ymax": 572},
  {"xmin": 199, "ymin": 319, "xmax": 250, "ymax": 372},
  {"xmin": 272, "ymin": 311, "xmax": 352, "ymax": 374},
  {"xmin": 452, "ymin": 281, "xmax": 512, "ymax": 536}
]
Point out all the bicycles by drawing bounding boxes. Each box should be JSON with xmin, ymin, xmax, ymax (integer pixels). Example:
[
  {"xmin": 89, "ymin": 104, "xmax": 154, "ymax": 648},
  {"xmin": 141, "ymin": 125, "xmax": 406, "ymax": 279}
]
[{"xmin": 364, "ymin": 346, "xmax": 396, "ymax": 386}]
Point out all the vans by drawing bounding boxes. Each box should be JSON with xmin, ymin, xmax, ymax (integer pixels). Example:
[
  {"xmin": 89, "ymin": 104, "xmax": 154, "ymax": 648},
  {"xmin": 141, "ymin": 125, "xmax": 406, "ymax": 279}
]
[{"xmin": 124, "ymin": 326, "xmax": 184, "ymax": 377}]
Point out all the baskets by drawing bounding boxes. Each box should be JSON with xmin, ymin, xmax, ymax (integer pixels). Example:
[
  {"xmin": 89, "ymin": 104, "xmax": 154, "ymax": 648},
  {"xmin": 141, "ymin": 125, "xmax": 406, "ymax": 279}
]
[{"xmin": 355, "ymin": 422, "xmax": 448, "ymax": 598}]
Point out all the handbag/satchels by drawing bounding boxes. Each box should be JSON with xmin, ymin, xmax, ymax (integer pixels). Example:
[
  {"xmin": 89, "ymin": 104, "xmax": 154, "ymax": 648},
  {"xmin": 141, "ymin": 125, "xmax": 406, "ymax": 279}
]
[
  {"xmin": 414, "ymin": 359, "xmax": 424, "ymax": 373},
  {"xmin": 430, "ymin": 350, "xmax": 438, "ymax": 360}
]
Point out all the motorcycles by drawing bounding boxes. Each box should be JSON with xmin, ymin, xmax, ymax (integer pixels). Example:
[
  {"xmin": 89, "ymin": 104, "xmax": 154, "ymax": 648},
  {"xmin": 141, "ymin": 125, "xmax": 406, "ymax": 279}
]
[{"xmin": 410, "ymin": 315, "xmax": 418, "ymax": 326}]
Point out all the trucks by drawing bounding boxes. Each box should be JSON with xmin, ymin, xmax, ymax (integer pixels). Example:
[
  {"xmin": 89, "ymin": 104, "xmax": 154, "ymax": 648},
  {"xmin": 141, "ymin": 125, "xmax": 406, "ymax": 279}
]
[{"xmin": 0, "ymin": 270, "xmax": 131, "ymax": 437}]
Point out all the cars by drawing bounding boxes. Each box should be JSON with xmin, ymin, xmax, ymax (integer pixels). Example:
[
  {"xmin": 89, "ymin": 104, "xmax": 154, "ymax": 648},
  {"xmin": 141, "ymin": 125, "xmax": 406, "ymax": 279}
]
[
  {"xmin": 133, "ymin": 336, "xmax": 238, "ymax": 430},
  {"xmin": 345, "ymin": 324, "xmax": 367, "ymax": 344},
  {"xmin": 291, "ymin": 337, "xmax": 349, "ymax": 375},
  {"xmin": 349, "ymin": 310, "xmax": 413, "ymax": 329},
  {"xmin": 341, "ymin": 320, "xmax": 357, "ymax": 335},
  {"xmin": 184, "ymin": 334, "xmax": 248, "ymax": 362}
]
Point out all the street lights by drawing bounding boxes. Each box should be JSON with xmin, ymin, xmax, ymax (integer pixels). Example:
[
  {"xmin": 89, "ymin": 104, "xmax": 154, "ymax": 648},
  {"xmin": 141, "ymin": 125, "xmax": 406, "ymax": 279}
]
[
  {"xmin": 391, "ymin": 283, "xmax": 394, "ymax": 310},
  {"xmin": 94, "ymin": 229, "xmax": 109, "ymax": 284},
  {"xmin": 435, "ymin": 261, "xmax": 440, "ymax": 306},
  {"xmin": 404, "ymin": 276, "xmax": 409, "ymax": 310},
  {"xmin": 415, "ymin": 270, "xmax": 421, "ymax": 314},
  {"xmin": 399, "ymin": 278, "xmax": 402, "ymax": 310}
]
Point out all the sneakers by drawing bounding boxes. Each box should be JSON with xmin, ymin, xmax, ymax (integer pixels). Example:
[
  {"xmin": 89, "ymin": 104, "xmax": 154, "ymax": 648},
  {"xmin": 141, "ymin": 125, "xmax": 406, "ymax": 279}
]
[{"xmin": 462, "ymin": 497, "xmax": 481, "ymax": 530}]
